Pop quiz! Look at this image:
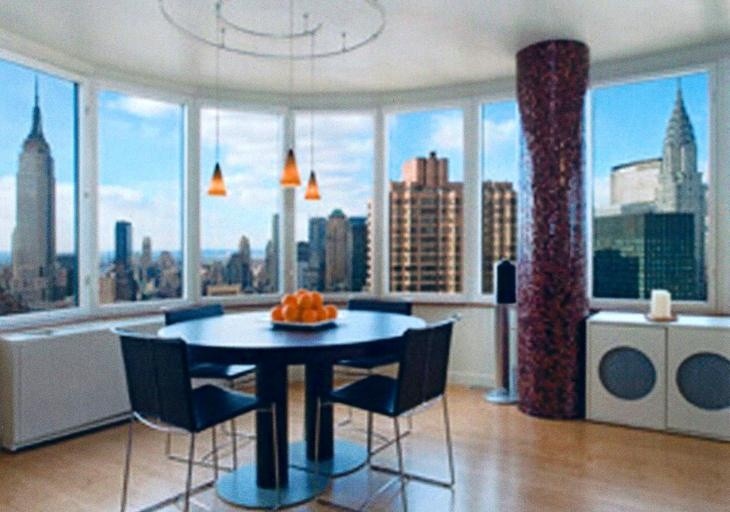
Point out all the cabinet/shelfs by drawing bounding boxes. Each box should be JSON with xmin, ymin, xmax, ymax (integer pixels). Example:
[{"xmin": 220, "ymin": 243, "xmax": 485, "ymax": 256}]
[
  {"xmin": 585, "ymin": 322, "xmax": 729, "ymax": 443},
  {"xmin": 0, "ymin": 319, "xmax": 167, "ymax": 450}
]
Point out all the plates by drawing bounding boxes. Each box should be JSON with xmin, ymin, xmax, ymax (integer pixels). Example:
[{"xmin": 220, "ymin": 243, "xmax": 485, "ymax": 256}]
[{"xmin": 269, "ymin": 318, "xmax": 338, "ymax": 329}]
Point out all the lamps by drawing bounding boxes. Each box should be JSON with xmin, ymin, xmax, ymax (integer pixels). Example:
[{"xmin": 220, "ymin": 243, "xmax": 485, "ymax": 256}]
[
  {"xmin": 207, "ymin": 0, "xmax": 228, "ymax": 198},
  {"xmin": 279, "ymin": 1, "xmax": 324, "ymax": 200}
]
